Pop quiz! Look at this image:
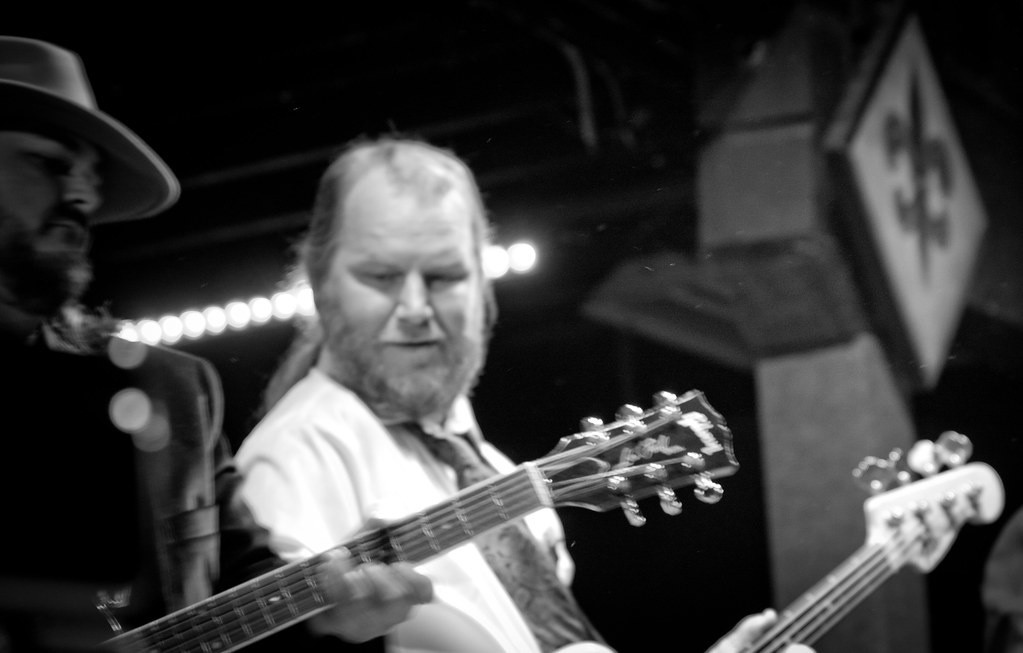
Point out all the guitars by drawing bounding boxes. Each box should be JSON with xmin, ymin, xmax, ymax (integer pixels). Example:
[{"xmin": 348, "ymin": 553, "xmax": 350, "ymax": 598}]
[
  {"xmin": 0, "ymin": 389, "xmax": 743, "ymax": 652},
  {"xmin": 709, "ymin": 428, "xmax": 1008, "ymax": 652}
]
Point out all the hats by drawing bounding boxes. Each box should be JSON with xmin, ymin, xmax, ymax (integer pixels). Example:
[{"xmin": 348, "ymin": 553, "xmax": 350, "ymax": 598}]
[{"xmin": 0, "ymin": 36, "xmax": 181, "ymax": 222}]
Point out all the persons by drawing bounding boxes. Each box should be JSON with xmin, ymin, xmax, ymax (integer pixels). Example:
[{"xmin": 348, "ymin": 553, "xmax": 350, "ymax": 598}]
[
  {"xmin": 0, "ymin": 34, "xmax": 433, "ymax": 653},
  {"xmin": 228, "ymin": 137, "xmax": 819, "ymax": 653}
]
